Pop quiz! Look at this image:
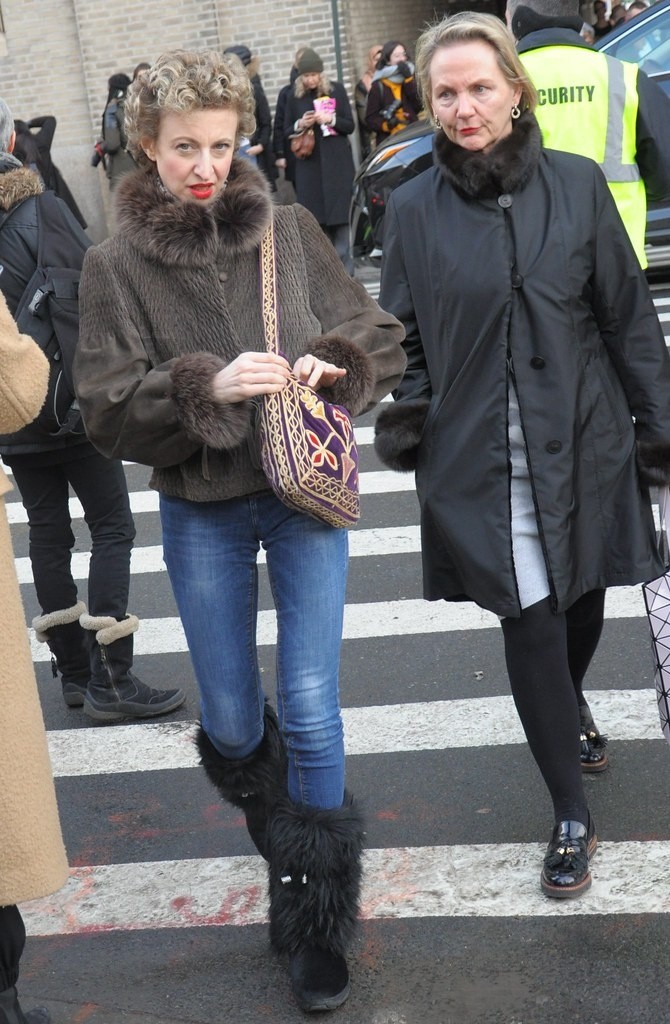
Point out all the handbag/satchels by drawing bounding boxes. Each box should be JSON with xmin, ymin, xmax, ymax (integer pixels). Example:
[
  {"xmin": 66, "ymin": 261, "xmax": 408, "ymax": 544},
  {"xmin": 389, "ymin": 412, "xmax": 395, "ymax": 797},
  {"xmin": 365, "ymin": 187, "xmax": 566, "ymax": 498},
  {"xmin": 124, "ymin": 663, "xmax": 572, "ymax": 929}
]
[
  {"xmin": 7, "ymin": 190, "xmax": 87, "ymax": 448},
  {"xmin": 249, "ymin": 373, "xmax": 363, "ymax": 533},
  {"xmin": 290, "ymin": 125, "xmax": 316, "ymax": 161},
  {"xmin": 640, "ymin": 565, "xmax": 670, "ymax": 742}
]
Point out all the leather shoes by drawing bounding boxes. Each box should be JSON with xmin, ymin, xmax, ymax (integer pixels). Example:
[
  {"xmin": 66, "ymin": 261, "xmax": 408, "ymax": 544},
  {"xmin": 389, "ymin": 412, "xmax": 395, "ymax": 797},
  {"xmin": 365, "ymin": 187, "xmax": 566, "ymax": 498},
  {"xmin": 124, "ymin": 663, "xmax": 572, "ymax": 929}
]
[
  {"xmin": 538, "ymin": 806, "xmax": 597, "ymax": 898},
  {"xmin": 573, "ymin": 701, "xmax": 609, "ymax": 775}
]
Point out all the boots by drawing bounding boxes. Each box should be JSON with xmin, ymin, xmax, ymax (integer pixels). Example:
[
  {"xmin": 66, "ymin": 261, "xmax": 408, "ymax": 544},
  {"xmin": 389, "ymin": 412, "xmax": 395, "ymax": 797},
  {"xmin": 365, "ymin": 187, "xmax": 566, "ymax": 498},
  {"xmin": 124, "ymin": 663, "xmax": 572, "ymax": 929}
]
[
  {"xmin": 75, "ymin": 614, "xmax": 185, "ymax": 719},
  {"xmin": 195, "ymin": 693, "xmax": 287, "ymax": 864},
  {"xmin": 262, "ymin": 790, "xmax": 363, "ymax": 1011},
  {"xmin": 1, "ymin": 989, "xmax": 49, "ymax": 1023},
  {"xmin": 30, "ymin": 603, "xmax": 96, "ymax": 706}
]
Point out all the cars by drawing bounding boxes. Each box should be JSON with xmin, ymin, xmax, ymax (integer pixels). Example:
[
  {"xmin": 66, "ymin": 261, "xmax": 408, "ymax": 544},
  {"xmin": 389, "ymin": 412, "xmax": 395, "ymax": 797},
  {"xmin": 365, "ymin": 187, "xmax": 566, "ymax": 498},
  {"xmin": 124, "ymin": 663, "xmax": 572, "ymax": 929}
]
[{"xmin": 345, "ymin": 1, "xmax": 670, "ymax": 269}]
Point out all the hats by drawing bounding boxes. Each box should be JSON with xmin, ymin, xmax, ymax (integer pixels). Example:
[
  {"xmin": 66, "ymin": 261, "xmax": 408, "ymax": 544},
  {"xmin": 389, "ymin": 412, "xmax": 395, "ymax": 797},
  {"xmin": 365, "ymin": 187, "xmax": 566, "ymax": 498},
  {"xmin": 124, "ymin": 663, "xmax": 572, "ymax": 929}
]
[
  {"xmin": 108, "ymin": 74, "xmax": 131, "ymax": 90},
  {"xmin": 296, "ymin": 49, "xmax": 322, "ymax": 74}
]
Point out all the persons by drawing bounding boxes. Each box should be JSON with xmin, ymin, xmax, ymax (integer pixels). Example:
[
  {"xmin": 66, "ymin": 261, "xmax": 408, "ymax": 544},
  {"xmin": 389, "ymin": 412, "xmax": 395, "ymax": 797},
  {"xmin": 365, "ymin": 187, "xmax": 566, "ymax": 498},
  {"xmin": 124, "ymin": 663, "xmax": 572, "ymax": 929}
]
[
  {"xmin": 273, "ymin": 48, "xmax": 356, "ymax": 280},
  {"xmin": 0, "ymin": 103, "xmax": 186, "ymax": 722},
  {"xmin": 476, "ymin": 1, "xmax": 668, "ymax": 354},
  {"xmin": 9, "ymin": 114, "xmax": 87, "ymax": 244},
  {"xmin": 74, "ymin": 42, "xmax": 410, "ymax": 1019},
  {"xmin": 91, "ymin": 71, "xmax": 136, "ymax": 192},
  {"xmin": 129, "ymin": 62, "xmax": 152, "ymax": 79},
  {"xmin": 1, "ymin": 274, "xmax": 79, "ymax": 1024},
  {"xmin": 350, "ymin": 45, "xmax": 395, "ymax": 257},
  {"xmin": 364, "ymin": 38, "xmax": 425, "ymax": 264},
  {"xmin": 221, "ymin": 44, "xmax": 274, "ymax": 194},
  {"xmin": 288, "ymin": 48, "xmax": 309, "ymax": 86},
  {"xmin": 361, "ymin": 10, "xmax": 669, "ymax": 904},
  {"xmin": 570, "ymin": 1, "xmax": 668, "ymax": 78}
]
